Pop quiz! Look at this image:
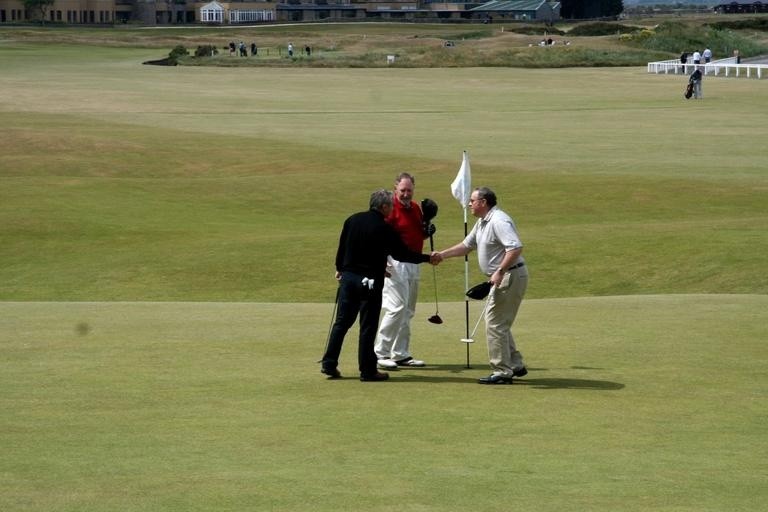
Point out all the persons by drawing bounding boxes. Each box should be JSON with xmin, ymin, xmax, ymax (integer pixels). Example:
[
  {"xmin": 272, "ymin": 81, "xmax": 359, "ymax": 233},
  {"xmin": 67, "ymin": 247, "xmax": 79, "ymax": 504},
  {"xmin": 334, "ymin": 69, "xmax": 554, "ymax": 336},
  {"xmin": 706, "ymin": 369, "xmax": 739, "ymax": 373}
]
[
  {"xmin": 320, "ymin": 189, "xmax": 444, "ymax": 382},
  {"xmin": 701, "ymin": 45, "xmax": 712, "ymax": 63},
  {"xmin": 733, "ymin": 48, "xmax": 741, "ymax": 64},
  {"xmin": 430, "ymin": 184, "xmax": 529, "ymax": 386},
  {"xmin": 372, "ymin": 172, "xmax": 436, "ymax": 371},
  {"xmin": 225, "ymin": 37, "xmax": 311, "ymax": 59},
  {"xmin": 689, "ymin": 69, "xmax": 702, "ymax": 100},
  {"xmin": 536, "ymin": 37, "xmax": 571, "ymax": 49},
  {"xmin": 681, "ymin": 53, "xmax": 687, "ymax": 73},
  {"xmin": 692, "ymin": 49, "xmax": 701, "ymax": 64}
]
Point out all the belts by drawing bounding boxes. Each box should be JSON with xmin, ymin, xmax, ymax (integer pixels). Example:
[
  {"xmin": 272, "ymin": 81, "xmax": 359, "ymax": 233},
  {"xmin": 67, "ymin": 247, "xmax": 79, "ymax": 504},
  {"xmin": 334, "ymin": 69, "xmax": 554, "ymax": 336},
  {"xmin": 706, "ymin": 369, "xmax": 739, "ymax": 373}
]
[{"xmin": 508, "ymin": 262, "xmax": 524, "ymax": 270}]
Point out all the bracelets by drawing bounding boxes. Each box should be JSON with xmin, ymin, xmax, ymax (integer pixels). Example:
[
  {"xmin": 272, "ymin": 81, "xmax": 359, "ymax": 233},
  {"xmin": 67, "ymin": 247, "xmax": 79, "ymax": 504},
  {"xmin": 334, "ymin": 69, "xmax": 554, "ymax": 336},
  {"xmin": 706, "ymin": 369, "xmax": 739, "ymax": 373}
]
[{"xmin": 497, "ymin": 267, "xmax": 505, "ymax": 274}]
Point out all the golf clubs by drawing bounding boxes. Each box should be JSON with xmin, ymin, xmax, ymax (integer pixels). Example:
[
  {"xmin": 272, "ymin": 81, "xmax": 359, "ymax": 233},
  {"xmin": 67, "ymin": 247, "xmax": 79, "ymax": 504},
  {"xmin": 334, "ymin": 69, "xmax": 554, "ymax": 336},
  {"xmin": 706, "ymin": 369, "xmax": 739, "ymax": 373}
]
[
  {"xmin": 428, "ymin": 235, "xmax": 442, "ymax": 323},
  {"xmin": 460, "ymin": 295, "xmax": 491, "ymax": 343},
  {"xmin": 318, "ymin": 287, "xmax": 340, "ymax": 363}
]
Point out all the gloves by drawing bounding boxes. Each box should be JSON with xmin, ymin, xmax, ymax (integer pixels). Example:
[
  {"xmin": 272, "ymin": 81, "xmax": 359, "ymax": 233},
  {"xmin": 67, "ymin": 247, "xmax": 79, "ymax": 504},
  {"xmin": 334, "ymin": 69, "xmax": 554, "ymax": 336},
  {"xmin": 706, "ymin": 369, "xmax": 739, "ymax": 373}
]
[
  {"xmin": 361, "ymin": 277, "xmax": 375, "ymax": 290},
  {"xmin": 423, "ymin": 224, "xmax": 436, "ymax": 239}
]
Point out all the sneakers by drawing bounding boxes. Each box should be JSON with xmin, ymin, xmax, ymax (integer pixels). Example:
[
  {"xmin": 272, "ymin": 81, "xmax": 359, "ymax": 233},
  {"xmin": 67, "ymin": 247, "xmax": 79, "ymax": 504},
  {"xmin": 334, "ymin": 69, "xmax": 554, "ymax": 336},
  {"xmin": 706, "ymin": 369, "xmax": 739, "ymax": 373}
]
[
  {"xmin": 360, "ymin": 372, "xmax": 388, "ymax": 381},
  {"xmin": 398, "ymin": 360, "xmax": 425, "ymax": 366},
  {"xmin": 377, "ymin": 359, "xmax": 397, "ymax": 368},
  {"xmin": 321, "ymin": 367, "xmax": 340, "ymax": 378}
]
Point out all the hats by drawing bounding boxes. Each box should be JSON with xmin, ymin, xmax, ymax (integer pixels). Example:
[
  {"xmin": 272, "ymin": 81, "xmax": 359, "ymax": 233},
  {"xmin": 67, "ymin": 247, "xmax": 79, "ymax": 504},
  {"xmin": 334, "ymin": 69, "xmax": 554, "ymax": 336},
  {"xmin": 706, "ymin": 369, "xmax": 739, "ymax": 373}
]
[
  {"xmin": 421, "ymin": 198, "xmax": 438, "ymax": 225},
  {"xmin": 465, "ymin": 281, "xmax": 494, "ymax": 300}
]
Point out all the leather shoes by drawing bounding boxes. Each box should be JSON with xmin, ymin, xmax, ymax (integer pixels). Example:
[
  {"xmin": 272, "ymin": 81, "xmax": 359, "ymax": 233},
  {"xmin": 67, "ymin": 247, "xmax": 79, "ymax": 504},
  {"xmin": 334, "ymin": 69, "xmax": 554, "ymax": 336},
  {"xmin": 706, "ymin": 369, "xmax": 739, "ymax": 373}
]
[
  {"xmin": 477, "ymin": 374, "xmax": 512, "ymax": 384},
  {"xmin": 512, "ymin": 366, "xmax": 527, "ymax": 377}
]
